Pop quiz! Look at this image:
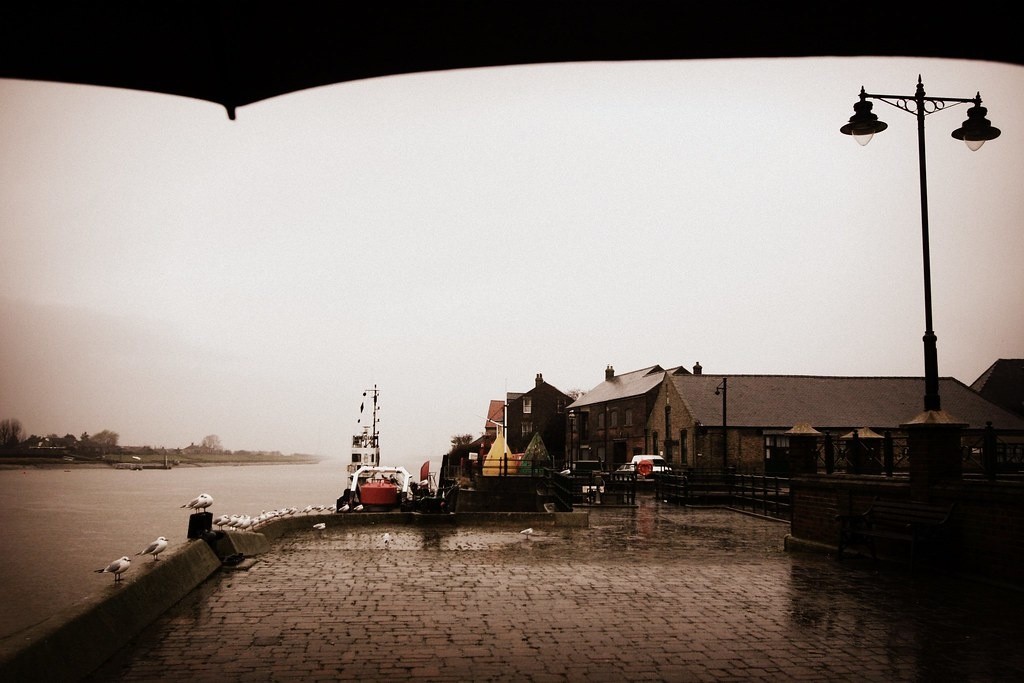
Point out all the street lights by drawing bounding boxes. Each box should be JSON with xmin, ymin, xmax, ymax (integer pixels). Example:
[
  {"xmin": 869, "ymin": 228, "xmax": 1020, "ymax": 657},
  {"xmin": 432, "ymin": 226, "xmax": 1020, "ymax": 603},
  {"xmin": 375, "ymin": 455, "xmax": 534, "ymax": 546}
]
[
  {"xmin": 714, "ymin": 386, "xmax": 727, "ymax": 475},
  {"xmin": 569, "ymin": 410, "xmax": 574, "ymax": 473},
  {"xmin": 839, "ymin": 73, "xmax": 1002, "ymax": 413}
]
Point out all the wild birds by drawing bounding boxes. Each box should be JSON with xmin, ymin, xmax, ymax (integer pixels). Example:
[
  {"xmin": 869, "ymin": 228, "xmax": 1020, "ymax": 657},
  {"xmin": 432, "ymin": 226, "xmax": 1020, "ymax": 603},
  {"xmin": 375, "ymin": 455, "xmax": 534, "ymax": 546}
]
[
  {"xmin": 383, "ymin": 532, "xmax": 390, "ymax": 545},
  {"xmin": 180, "ymin": 493, "xmax": 213, "ymax": 514},
  {"xmin": 134, "ymin": 536, "xmax": 169, "ymax": 562},
  {"xmin": 93, "ymin": 556, "xmax": 132, "ymax": 581},
  {"xmin": 212, "ymin": 502, "xmax": 363, "ymax": 534},
  {"xmin": 520, "ymin": 527, "xmax": 533, "ymax": 540}
]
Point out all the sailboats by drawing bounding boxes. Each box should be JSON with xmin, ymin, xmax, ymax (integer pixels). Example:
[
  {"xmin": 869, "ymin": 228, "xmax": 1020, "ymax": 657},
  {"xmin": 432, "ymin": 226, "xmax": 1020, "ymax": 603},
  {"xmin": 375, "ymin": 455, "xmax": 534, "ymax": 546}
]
[{"xmin": 345, "ymin": 384, "xmax": 412, "ymax": 501}]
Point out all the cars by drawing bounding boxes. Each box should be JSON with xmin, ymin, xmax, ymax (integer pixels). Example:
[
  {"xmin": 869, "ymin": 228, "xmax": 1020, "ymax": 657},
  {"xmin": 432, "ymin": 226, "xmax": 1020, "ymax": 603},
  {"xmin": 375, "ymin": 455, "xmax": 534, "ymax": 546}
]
[{"xmin": 616, "ymin": 462, "xmax": 631, "ymax": 471}]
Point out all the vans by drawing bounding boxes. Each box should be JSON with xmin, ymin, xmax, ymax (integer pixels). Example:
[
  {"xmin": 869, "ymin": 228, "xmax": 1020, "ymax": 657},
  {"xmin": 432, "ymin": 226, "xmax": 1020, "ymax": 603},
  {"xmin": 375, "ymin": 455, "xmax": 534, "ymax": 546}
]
[{"xmin": 630, "ymin": 455, "xmax": 672, "ymax": 472}]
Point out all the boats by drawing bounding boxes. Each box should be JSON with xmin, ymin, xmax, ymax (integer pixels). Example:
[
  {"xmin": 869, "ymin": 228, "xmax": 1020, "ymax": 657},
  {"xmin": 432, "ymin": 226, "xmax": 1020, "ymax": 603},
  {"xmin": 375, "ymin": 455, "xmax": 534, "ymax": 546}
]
[
  {"xmin": 405, "ymin": 461, "xmax": 437, "ymax": 499},
  {"xmin": 130, "ymin": 465, "xmax": 142, "ymax": 471}
]
